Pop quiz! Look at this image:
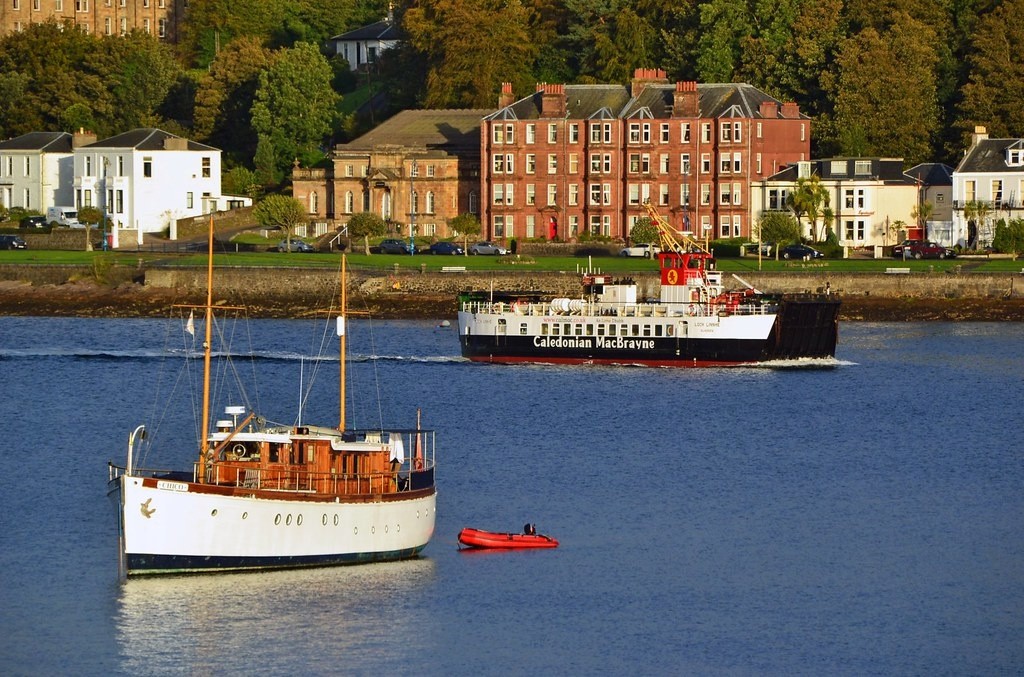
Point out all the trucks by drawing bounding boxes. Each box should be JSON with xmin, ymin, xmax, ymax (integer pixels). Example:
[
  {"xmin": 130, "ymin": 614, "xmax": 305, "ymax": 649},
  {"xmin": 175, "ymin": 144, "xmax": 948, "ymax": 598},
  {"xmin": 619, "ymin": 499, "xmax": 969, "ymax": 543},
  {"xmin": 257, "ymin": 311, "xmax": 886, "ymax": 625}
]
[{"xmin": 46, "ymin": 206, "xmax": 79, "ymax": 228}]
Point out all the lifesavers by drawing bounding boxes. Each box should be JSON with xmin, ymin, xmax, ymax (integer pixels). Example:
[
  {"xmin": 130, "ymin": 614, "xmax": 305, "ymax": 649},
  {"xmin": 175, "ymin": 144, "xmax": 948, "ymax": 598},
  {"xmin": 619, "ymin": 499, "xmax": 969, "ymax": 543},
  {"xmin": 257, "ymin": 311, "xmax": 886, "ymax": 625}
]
[
  {"xmin": 493, "ymin": 303, "xmax": 500, "ymax": 313},
  {"xmin": 688, "ymin": 304, "xmax": 696, "ymax": 315},
  {"xmin": 414, "ymin": 457, "xmax": 422, "ymax": 469},
  {"xmin": 668, "ymin": 326, "xmax": 671, "ymax": 335}
]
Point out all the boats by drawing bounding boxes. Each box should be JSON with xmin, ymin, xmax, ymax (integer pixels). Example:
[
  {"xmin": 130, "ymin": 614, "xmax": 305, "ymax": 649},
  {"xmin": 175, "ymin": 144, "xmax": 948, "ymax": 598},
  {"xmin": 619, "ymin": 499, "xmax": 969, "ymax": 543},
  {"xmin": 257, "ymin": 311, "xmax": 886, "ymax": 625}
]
[
  {"xmin": 107, "ymin": 216, "xmax": 436, "ymax": 580},
  {"xmin": 457, "ymin": 523, "xmax": 560, "ymax": 548},
  {"xmin": 459, "ymin": 165, "xmax": 843, "ymax": 368}
]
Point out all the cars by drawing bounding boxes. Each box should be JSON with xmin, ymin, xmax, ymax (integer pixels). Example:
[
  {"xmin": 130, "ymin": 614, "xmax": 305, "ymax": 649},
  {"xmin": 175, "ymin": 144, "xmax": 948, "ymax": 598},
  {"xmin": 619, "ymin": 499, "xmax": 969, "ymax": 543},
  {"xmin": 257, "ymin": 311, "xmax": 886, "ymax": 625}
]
[
  {"xmin": 619, "ymin": 244, "xmax": 661, "ymax": 259},
  {"xmin": 0, "ymin": 235, "xmax": 28, "ymax": 249},
  {"xmin": 278, "ymin": 238, "xmax": 315, "ymax": 253},
  {"xmin": 893, "ymin": 239, "xmax": 957, "ymax": 259},
  {"xmin": 470, "ymin": 242, "xmax": 507, "ymax": 256},
  {"xmin": 429, "ymin": 242, "xmax": 464, "ymax": 255},
  {"xmin": 783, "ymin": 245, "xmax": 824, "ymax": 259},
  {"xmin": 19, "ymin": 216, "xmax": 46, "ymax": 227},
  {"xmin": 379, "ymin": 239, "xmax": 421, "ymax": 255}
]
